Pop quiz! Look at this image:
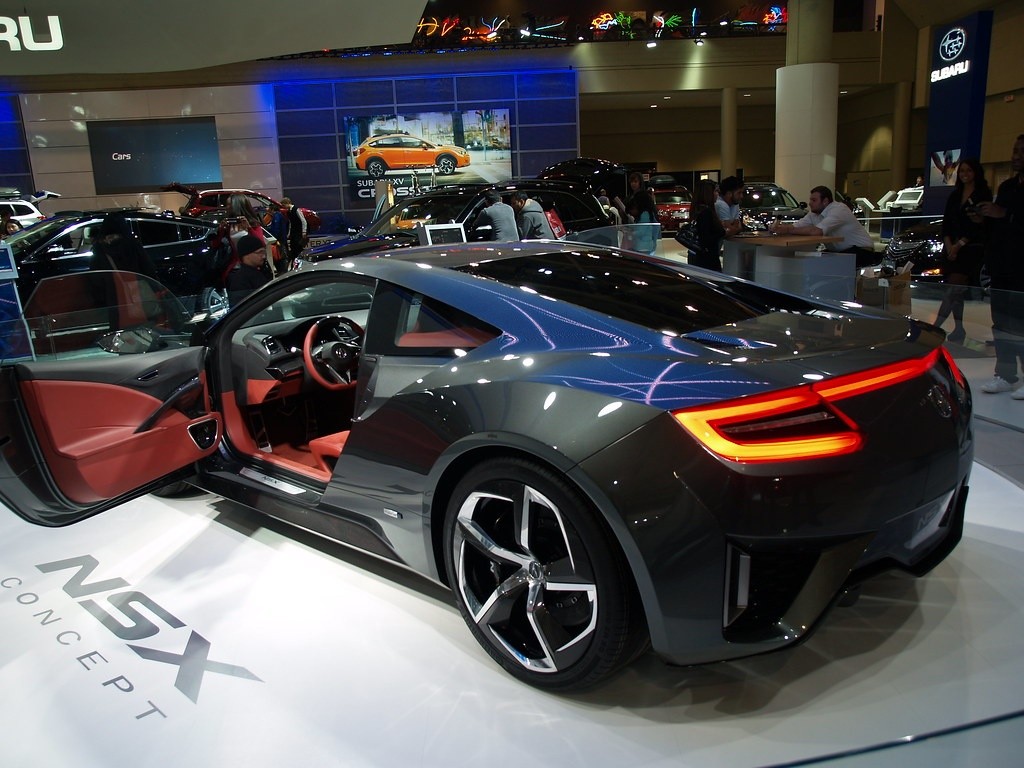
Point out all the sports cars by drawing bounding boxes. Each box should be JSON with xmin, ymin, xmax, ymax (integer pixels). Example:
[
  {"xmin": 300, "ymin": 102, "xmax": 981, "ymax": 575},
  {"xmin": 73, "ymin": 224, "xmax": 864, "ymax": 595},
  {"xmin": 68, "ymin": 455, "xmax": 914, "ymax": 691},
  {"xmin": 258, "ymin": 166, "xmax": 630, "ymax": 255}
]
[{"xmin": 0, "ymin": 235, "xmax": 973, "ymax": 688}]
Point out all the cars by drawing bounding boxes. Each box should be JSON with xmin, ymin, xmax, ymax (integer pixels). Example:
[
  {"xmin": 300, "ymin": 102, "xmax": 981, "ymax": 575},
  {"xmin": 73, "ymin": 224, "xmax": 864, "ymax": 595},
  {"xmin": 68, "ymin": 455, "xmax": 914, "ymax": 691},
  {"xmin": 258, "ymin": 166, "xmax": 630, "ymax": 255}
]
[
  {"xmin": 883, "ymin": 204, "xmax": 991, "ymax": 297},
  {"xmin": 397, "ymin": 206, "xmax": 435, "ymax": 232},
  {"xmin": 0, "ymin": 207, "xmax": 288, "ymax": 358}
]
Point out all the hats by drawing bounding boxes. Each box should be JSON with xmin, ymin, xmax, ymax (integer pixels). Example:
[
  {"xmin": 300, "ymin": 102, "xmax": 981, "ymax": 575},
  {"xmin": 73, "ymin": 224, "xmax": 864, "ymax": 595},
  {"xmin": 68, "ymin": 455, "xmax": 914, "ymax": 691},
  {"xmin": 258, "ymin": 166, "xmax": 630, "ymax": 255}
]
[{"xmin": 237, "ymin": 235, "xmax": 265, "ymax": 257}]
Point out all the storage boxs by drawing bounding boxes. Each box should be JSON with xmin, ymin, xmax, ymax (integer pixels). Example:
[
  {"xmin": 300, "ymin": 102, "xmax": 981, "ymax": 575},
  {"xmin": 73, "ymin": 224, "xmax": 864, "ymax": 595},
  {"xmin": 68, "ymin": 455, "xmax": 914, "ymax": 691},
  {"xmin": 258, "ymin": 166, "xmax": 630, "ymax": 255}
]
[{"xmin": 857, "ymin": 271, "xmax": 911, "ymax": 314}]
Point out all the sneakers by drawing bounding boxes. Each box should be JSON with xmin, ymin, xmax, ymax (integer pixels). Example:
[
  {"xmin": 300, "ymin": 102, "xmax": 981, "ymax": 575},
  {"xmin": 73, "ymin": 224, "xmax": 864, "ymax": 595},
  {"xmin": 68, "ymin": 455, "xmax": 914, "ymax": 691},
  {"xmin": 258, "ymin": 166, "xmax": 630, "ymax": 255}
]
[
  {"xmin": 981, "ymin": 376, "xmax": 1012, "ymax": 393},
  {"xmin": 1012, "ymin": 386, "xmax": 1024, "ymax": 399}
]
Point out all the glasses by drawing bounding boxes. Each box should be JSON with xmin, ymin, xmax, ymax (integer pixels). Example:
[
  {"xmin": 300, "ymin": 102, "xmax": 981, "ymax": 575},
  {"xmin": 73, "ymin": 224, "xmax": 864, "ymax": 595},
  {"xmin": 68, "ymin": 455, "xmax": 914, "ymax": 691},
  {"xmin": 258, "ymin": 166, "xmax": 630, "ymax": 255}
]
[{"xmin": 253, "ymin": 251, "xmax": 266, "ymax": 256}]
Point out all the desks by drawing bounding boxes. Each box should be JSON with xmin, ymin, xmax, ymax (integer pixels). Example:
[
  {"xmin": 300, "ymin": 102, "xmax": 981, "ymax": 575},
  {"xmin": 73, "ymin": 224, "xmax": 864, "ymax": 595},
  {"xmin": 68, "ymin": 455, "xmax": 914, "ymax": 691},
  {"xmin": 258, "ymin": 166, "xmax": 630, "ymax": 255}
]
[{"xmin": 723, "ymin": 234, "xmax": 845, "ymax": 287}]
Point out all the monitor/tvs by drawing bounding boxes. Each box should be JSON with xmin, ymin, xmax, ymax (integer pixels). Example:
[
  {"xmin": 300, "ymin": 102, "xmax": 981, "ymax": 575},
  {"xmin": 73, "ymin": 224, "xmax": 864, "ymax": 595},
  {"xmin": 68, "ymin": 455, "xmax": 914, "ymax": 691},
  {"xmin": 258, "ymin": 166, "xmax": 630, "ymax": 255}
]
[
  {"xmin": 877, "ymin": 190, "xmax": 924, "ymax": 205},
  {"xmin": 855, "ymin": 198, "xmax": 878, "ymax": 211}
]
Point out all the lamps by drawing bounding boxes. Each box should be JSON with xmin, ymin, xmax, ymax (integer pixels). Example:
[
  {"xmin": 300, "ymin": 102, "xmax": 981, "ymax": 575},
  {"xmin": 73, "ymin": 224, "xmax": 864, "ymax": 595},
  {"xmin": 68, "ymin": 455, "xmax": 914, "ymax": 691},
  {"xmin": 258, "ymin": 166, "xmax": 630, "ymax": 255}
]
[
  {"xmin": 462, "ymin": 32, "xmax": 498, "ymax": 39},
  {"xmin": 693, "ymin": 36, "xmax": 704, "ymax": 47}
]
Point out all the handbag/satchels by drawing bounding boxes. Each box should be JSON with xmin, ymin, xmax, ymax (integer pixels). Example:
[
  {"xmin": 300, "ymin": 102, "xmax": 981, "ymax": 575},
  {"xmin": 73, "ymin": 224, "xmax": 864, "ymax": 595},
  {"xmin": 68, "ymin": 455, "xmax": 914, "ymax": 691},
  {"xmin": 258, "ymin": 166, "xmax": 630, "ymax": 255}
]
[{"xmin": 676, "ymin": 207, "xmax": 709, "ymax": 255}]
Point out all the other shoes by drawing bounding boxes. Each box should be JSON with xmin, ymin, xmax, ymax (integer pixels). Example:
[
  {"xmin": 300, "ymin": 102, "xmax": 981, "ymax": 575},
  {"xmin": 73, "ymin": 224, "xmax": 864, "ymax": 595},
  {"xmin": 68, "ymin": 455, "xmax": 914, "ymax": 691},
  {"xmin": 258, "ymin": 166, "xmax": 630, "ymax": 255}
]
[{"xmin": 947, "ymin": 328, "xmax": 966, "ymax": 341}]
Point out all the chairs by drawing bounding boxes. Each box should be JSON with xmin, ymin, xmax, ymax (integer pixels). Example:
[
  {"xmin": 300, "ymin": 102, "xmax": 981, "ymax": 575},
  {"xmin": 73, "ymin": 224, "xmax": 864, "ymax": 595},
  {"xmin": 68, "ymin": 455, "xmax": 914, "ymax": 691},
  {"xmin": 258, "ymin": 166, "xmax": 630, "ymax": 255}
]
[
  {"xmin": 308, "ymin": 425, "xmax": 349, "ymax": 476},
  {"xmin": 78, "ymin": 227, "xmax": 94, "ymax": 253}
]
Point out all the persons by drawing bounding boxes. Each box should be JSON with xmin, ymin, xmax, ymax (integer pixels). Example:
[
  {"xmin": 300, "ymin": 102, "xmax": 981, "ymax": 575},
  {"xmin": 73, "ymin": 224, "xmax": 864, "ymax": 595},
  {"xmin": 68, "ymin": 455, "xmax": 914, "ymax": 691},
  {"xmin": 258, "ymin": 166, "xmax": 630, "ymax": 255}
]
[
  {"xmin": 714, "ymin": 177, "xmax": 745, "ymax": 233},
  {"xmin": 225, "ymin": 235, "xmax": 284, "ymax": 329},
  {"xmin": 88, "ymin": 212, "xmax": 163, "ymax": 334},
  {"xmin": 598, "ymin": 172, "xmax": 662, "ymax": 257},
  {"xmin": 179, "ymin": 207, "xmax": 193, "ymax": 217},
  {"xmin": 510, "ymin": 191, "xmax": 556, "ymax": 241},
  {"xmin": 470, "ymin": 190, "xmax": 519, "ymax": 241},
  {"xmin": 768, "ymin": 185, "xmax": 875, "ymax": 271},
  {"xmin": 58, "ymin": 233, "xmax": 73, "ymax": 248},
  {"xmin": 210, "ymin": 193, "xmax": 266, "ymax": 279},
  {"xmin": 269, "ymin": 203, "xmax": 288, "ymax": 250},
  {"xmin": 281, "ymin": 197, "xmax": 310, "ymax": 259},
  {"xmin": 934, "ymin": 158, "xmax": 993, "ymax": 340},
  {"xmin": 688, "ymin": 179, "xmax": 737, "ymax": 273},
  {"xmin": 965, "ymin": 134, "xmax": 1024, "ymax": 400},
  {"xmin": 914, "ymin": 176, "xmax": 925, "ymax": 187},
  {"xmin": 448, "ymin": 7, "xmax": 735, "ymax": 46},
  {"xmin": 0, "ymin": 206, "xmax": 23, "ymax": 239}
]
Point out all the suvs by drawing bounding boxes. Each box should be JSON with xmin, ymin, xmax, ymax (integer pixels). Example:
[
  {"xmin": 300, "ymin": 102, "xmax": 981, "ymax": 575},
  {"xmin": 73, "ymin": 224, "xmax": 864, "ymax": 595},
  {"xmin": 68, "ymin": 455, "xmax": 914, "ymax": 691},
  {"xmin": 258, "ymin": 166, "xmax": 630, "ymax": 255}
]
[
  {"xmin": 355, "ymin": 131, "xmax": 470, "ymax": 176},
  {"xmin": 536, "ymin": 158, "xmax": 632, "ymax": 199},
  {"xmin": 648, "ymin": 176, "xmax": 694, "ymax": 230},
  {"xmin": 0, "ymin": 186, "xmax": 61, "ymax": 237},
  {"xmin": 740, "ymin": 182, "xmax": 809, "ymax": 233},
  {"xmin": 290, "ymin": 182, "xmax": 625, "ymax": 309},
  {"xmin": 160, "ymin": 182, "xmax": 321, "ymax": 243}
]
